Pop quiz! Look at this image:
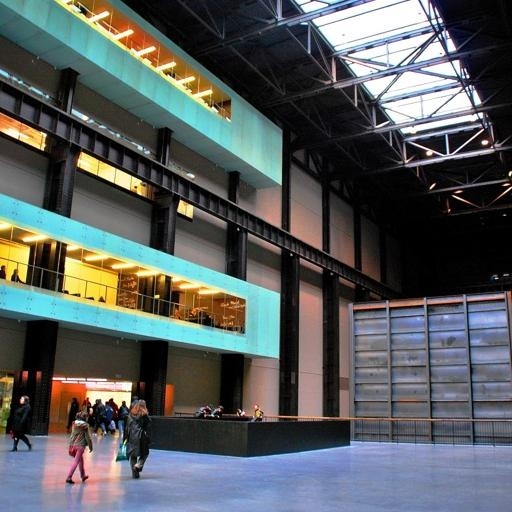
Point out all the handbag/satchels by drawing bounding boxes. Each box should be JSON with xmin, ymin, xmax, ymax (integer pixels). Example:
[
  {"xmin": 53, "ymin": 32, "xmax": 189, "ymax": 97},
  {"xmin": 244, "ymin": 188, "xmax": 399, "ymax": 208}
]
[
  {"xmin": 115, "ymin": 438, "xmax": 129, "ymax": 463},
  {"xmin": 68, "ymin": 444, "xmax": 78, "ymax": 456},
  {"xmin": 9, "ymin": 430, "xmax": 14, "ymax": 439}
]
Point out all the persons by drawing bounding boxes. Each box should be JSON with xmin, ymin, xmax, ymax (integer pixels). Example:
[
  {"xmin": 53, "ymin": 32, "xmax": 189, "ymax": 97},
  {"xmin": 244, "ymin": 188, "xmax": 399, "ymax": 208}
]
[
  {"xmin": 11, "ymin": 269, "xmax": 25, "ymax": 284},
  {"xmin": 0, "ymin": 265, "xmax": 6, "ymax": 279},
  {"xmin": 8, "ymin": 395, "xmax": 34, "ymax": 452},
  {"xmin": 211, "ymin": 406, "xmax": 224, "ymax": 418},
  {"xmin": 250, "ymin": 404, "xmax": 264, "ymax": 422},
  {"xmin": 66, "ymin": 396, "xmax": 140, "ymax": 438},
  {"xmin": 195, "ymin": 406, "xmax": 213, "ymax": 418},
  {"xmin": 66, "ymin": 410, "xmax": 94, "ymax": 484},
  {"xmin": 125, "ymin": 399, "xmax": 153, "ymax": 479},
  {"xmin": 236, "ymin": 409, "xmax": 246, "ymax": 417}
]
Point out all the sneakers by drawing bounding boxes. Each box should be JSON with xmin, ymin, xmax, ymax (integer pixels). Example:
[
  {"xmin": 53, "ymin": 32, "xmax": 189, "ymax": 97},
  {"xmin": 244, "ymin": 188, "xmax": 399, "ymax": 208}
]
[
  {"xmin": 10, "ymin": 443, "xmax": 32, "ymax": 452},
  {"xmin": 132, "ymin": 463, "xmax": 143, "ymax": 479},
  {"xmin": 65, "ymin": 476, "xmax": 76, "ymax": 485},
  {"xmin": 82, "ymin": 475, "xmax": 89, "ymax": 482}
]
